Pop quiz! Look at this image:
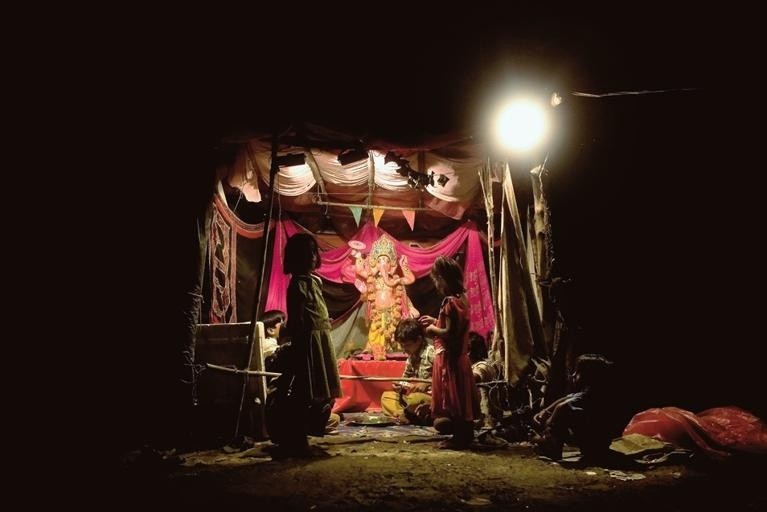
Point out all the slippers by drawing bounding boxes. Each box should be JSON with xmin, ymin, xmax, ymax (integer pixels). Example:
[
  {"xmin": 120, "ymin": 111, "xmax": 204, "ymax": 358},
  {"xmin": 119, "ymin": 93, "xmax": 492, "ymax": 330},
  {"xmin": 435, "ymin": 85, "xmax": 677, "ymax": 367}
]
[{"xmin": 224, "ymin": 435, "xmax": 254, "ymax": 452}]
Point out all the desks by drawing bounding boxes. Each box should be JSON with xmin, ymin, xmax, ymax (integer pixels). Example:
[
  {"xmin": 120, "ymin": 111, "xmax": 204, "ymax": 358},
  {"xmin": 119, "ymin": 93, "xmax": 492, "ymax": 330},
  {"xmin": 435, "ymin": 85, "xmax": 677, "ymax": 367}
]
[{"xmin": 338, "ymin": 360, "xmax": 407, "ymax": 411}]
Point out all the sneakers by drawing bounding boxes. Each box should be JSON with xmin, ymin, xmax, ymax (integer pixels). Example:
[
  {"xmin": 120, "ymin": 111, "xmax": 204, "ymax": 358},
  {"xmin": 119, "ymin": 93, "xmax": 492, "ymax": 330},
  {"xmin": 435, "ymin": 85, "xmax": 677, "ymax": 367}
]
[{"xmin": 478, "ymin": 430, "xmax": 507, "ymax": 447}]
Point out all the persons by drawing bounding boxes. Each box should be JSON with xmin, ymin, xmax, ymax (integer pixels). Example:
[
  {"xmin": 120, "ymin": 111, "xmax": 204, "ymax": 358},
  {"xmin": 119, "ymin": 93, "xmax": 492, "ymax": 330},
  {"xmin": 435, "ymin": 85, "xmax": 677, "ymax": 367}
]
[
  {"xmin": 532, "ymin": 351, "xmax": 624, "ymax": 462},
  {"xmin": 418, "ymin": 255, "xmax": 483, "ymax": 449},
  {"xmin": 433, "ymin": 331, "xmax": 495, "ymax": 435},
  {"xmin": 275, "ymin": 232, "xmax": 341, "ymax": 457},
  {"xmin": 381, "ymin": 316, "xmax": 436, "ymax": 425},
  {"xmin": 257, "ymin": 309, "xmax": 341, "ymax": 433}
]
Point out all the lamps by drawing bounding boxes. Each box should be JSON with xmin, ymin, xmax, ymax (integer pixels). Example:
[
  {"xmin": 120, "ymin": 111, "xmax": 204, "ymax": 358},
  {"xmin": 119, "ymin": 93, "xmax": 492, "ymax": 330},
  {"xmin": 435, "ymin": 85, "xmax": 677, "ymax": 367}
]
[
  {"xmin": 273, "ymin": 151, "xmax": 307, "ymax": 169},
  {"xmin": 337, "ymin": 143, "xmax": 369, "ymax": 166}
]
